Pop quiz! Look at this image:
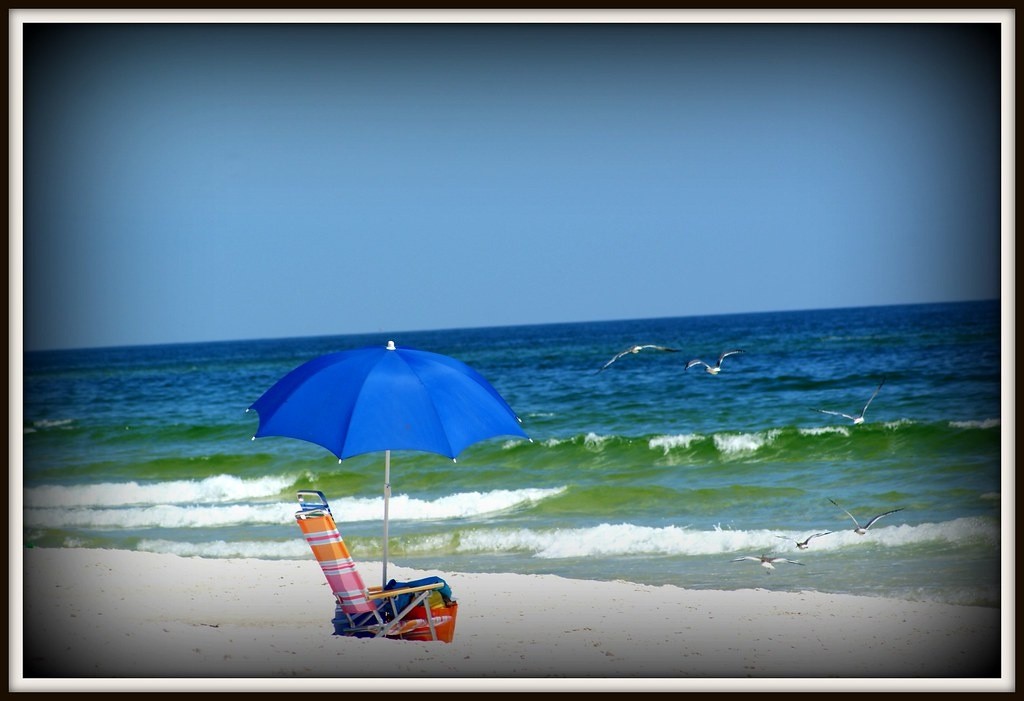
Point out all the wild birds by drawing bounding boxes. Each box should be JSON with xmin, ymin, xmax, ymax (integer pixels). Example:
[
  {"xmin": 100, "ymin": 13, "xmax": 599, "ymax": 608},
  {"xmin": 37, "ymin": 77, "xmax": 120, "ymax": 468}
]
[
  {"xmin": 595, "ymin": 345, "xmax": 681, "ymax": 375},
  {"xmin": 829, "ymin": 500, "xmax": 906, "ymax": 534},
  {"xmin": 775, "ymin": 531, "xmax": 833, "ymax": 549},
  {"xmin": 732, "ymin": 553, "xmax": 807, "ymax": 570},
  {"xmin": 684, "ymin": 350, "xmax": 746, "ymax": 373},
  {"xmin": 810, "ymin": 377, "xmax": 888, "ymax": 425}
]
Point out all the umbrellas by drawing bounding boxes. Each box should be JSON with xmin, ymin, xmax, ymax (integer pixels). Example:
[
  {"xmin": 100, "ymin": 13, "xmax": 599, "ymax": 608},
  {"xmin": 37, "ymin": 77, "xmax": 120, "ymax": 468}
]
[{"xmin": 245, "ymin": 341, "xmax": 534, "ymax": 587}]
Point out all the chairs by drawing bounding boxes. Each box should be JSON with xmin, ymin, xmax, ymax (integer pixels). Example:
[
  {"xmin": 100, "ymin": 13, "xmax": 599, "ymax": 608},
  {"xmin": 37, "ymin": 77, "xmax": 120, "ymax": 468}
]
[{"xmin": 297, "ymin": 491, "xmax": 443, "ymax": 643}]
